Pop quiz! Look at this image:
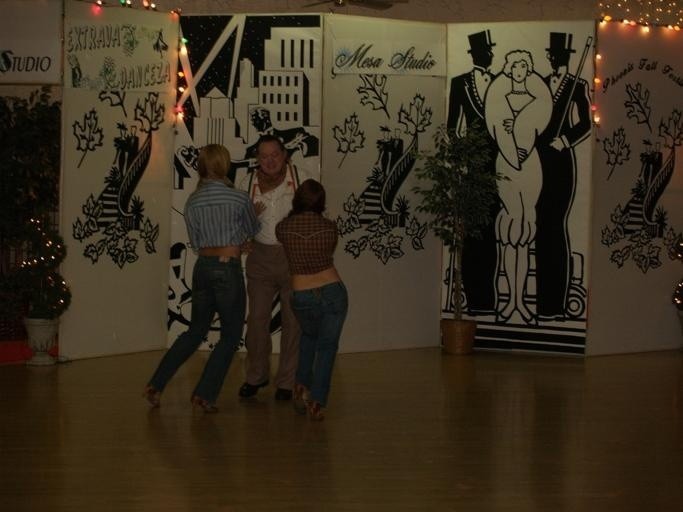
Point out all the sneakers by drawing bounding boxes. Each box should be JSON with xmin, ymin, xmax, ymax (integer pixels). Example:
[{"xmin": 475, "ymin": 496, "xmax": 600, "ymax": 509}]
[
  {"xmin": 275, "ymin": 388, "xmax": 290, "ymax": 401},
  {"xmin": 239, "ymin": 379, "xmax": 269, "ymax": 397}
]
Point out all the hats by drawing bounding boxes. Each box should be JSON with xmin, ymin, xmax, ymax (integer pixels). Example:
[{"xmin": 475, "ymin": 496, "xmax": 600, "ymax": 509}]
[
  {"xmin": 467, "ymin": 29, "xmax": 496, "ymax": 53},
  {"xmin": 545, "ymin": 32, "xmax": 576, "ymax": 53}
]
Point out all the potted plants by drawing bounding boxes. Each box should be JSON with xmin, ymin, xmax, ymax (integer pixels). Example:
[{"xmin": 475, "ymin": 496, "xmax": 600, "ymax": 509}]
[
  {"xmin": 0, "ymin": 81, "xmax": 73, "ymax": 366},
  {"xmin": 409, "ymin": 117, "xmax": 513, "ymax": 356}
]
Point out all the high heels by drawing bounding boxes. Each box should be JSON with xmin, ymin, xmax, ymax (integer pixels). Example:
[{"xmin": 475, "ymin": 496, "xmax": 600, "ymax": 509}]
[
  {"xmin": 292, "ymin": 389, "xmax": 324, "ymax": 420},
  {"xmin": 190, "ymin": 394, "xmax": 217, "ymax": 414},
  {"xmin": 142, "ymin": 385, "xmax": 162, "ymax": 407}
]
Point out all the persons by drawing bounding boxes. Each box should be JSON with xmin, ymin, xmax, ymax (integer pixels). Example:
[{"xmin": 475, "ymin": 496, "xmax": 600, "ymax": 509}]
[
  {"xmin": 234, "ymin": 132, "xmax": 313, "ymax": 403},
  {"xmin": 534, "ymin": 29, "xmax": 592, "ymax": 322},
  {"xmin": 273, "ymin": 178, "xmax": 350, "ymax": 425},
  {"xmin": 478, "ymin": 49, "xmax": 554, "ymax": 328},
  {"xmin": 437, "ymin": 28, "xmax": 501, "ymax": 314},
  {"xmin": 138, "ymin": 144, "xmax": 266, "ymax": 415}
]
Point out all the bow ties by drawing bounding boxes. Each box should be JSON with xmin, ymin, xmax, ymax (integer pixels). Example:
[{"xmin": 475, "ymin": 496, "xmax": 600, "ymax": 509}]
[
  {"xmin": 481, "ymin": 68, "xmax": 491, "ymax": 76},
  {"xmin": 552, "ymin": 71, "xmax": 562, "ymax": 79}
]
